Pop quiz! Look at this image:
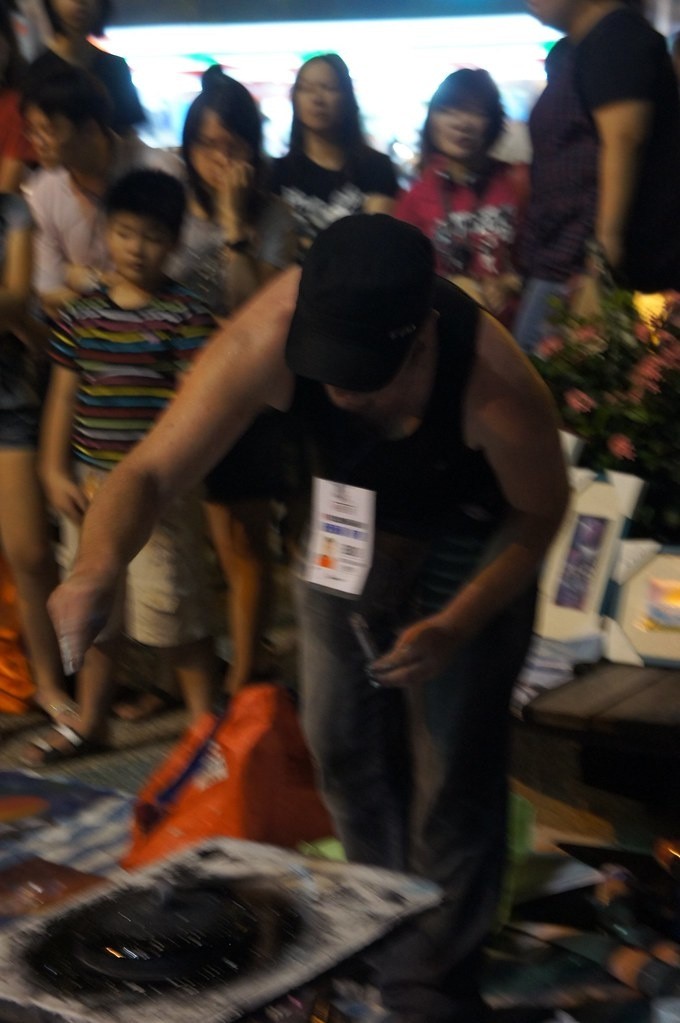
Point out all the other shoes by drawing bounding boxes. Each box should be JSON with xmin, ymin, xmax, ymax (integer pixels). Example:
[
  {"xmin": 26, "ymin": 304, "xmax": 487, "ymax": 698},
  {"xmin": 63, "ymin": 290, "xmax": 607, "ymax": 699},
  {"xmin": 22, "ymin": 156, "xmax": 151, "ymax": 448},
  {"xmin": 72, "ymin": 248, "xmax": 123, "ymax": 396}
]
[{"xmin": 112, "ymin": 687, "xmax": 183, "ymax": 718}]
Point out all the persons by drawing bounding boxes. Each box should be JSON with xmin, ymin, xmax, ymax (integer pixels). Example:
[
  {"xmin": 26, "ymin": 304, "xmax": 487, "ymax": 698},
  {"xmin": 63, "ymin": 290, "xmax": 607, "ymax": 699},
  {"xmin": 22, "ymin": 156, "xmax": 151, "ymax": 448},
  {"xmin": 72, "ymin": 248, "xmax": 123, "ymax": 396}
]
[
  {"xmin": 1, "ymin": 193, "xmax": 82, "ymax": 726},
  {"xmin": 17, "ymin": 166, "xmax": 222, "ymax": 771},
  {"xmin": 46, "ymin": 211, "xmax": 572, "ymax": 1022},
  {"xmin": 1, "ymin": 1, "xmax": 529, "ymax": 323},
  {"xmin": 511, "ymin": 0, "xmax": 680, "ymax": 353},
  {"xmin": 317, "ymin": 536, "xmax": 336, "ymax": 568}
]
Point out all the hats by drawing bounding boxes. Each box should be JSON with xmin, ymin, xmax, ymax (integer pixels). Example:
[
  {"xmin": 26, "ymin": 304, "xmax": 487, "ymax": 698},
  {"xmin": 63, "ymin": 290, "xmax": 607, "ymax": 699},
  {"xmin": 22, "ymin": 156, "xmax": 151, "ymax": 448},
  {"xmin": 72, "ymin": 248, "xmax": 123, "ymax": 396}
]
[{"xmin": 285, "ymin": 215, "xmax": 434, "ymax": 393}]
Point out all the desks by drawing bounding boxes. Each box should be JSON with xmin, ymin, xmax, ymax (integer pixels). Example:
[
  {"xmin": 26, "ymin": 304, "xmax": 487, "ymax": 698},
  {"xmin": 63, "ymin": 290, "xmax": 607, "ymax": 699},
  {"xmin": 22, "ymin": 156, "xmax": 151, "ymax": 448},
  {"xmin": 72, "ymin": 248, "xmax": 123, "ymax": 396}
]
[{"xmin": 523, "ymin": 661, "xmax": 679, "ymax": 833}]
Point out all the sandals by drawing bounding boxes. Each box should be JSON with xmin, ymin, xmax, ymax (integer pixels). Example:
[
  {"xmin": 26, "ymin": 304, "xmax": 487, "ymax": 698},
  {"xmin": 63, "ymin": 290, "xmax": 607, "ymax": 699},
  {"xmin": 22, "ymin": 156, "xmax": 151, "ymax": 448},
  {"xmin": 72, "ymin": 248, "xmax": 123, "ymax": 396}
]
[{"xmin": 18, "ymin": 724, "xmax": 99, "ymax": 767}]
